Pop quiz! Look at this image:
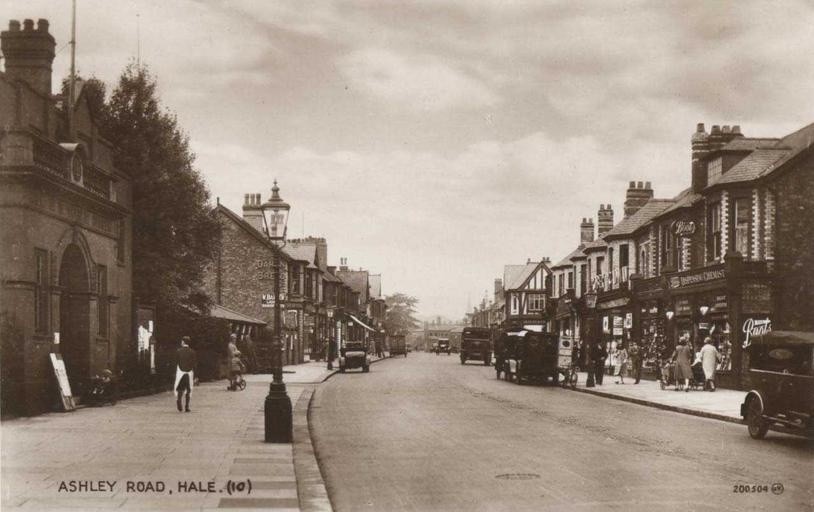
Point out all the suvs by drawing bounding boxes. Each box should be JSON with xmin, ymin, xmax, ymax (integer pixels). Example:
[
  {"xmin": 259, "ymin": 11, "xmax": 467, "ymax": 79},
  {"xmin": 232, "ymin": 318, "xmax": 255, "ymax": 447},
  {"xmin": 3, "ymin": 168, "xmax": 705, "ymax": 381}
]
[
  {"xmin": 740, "ymin": 331, "xmax": 814, "ymax": 441},
  {"xmin": 435, "ymin": 338, "xmax": 452, "ymax": 355},
  {"xmin": 338, "ymin": 342, "xmax": 369, "ymax": 372}
]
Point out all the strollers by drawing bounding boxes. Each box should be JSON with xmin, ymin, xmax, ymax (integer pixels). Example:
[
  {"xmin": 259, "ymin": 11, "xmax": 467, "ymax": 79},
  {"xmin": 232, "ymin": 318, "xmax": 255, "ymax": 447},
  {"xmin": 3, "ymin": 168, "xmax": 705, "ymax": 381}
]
[
  {"xmin": 686, "ymin": 361, "xmax": 709, "ymax": 392},
  {"xmin": 661, "ymin": 360, "xmax": 685, "ymax": 392}
]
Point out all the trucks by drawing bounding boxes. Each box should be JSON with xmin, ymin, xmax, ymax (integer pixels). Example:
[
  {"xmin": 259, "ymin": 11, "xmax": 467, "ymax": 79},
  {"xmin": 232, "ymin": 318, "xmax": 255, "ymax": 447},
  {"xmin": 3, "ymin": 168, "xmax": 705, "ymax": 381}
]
[
  {"xmin": 388, "ymin": 334, "xmax": 406, "ymax": 358},
  {"xmin": 458, "ymin": 327, "xmax": 492, "ymax": 366}
]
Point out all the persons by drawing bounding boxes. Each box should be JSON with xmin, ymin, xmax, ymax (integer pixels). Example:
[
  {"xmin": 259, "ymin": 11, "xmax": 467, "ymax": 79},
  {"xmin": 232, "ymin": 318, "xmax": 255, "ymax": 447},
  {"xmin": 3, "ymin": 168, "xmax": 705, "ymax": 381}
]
[
  {"xmin": 92, "ymin": 368, "xmax": 112, "ymax": 394},
  {"xmin": 231, "ymin": 351, "xmax": 245, "ymax": 384},
  {"xmin": 700, "ymin": 337, "xmax": 721, "ymax": 392},
  {"xmin": 628, "ymin": 342, "xmax": 642, "ymax": 384},
  {"xmin": 173, "ymin": 335, "xmax": 195, "ymax": 412},
  {"xmin": 670, "ymin": 337, "xmax": 693, "ymax": 392},
  {"xmin": 591, "ymin": 344, "xmax": 607, "ymax": 384},
  {"xmin": 613, "ymin": 343, "xmax": 628, "ymax": 384},
  {"xmin": 493, "ymin": 332, "xmax": 508, "ymax": 372},
  {"xmin": 228, "ymin": 332, "xmax": 245, "ymax": 389}
]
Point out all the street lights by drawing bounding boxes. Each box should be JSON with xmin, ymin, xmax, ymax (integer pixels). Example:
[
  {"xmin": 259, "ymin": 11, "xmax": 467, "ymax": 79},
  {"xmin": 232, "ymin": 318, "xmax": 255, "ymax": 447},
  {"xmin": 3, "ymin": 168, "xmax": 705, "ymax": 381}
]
[
  {"xmin": 323, "ymin": 302, "xmax": 338, "ymax": 371},
  {"xmin": 585, "ymin": 284, "xmax": 598, "ymax": 314},
  {"xmin": 258, "ymin": 179, "xmax": 295, "ymax": 443}
]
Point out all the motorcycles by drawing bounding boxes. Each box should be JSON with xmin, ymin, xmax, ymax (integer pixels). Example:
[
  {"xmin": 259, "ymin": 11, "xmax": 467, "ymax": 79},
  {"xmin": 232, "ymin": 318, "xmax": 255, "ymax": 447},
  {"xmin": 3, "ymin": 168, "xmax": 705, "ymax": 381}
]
[{"xmin": 86, "ymin": 368, "xmax": 125, "ymax": 408}]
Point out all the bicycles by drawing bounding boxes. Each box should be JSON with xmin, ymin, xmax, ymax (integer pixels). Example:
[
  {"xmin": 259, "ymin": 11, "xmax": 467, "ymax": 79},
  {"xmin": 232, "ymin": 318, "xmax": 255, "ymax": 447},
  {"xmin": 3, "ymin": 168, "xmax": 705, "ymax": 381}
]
[{"xmin": 228, "ymin": 367, "xmax": 246, "ymax": 391}]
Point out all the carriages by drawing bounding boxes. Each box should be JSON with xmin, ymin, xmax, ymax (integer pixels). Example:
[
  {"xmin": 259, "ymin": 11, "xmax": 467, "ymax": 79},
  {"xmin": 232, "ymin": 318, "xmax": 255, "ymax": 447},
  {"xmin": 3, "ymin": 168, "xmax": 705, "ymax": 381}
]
[{"xmin": 494, "ymin": 326, "xmax": 561, "ymax": 386}]
